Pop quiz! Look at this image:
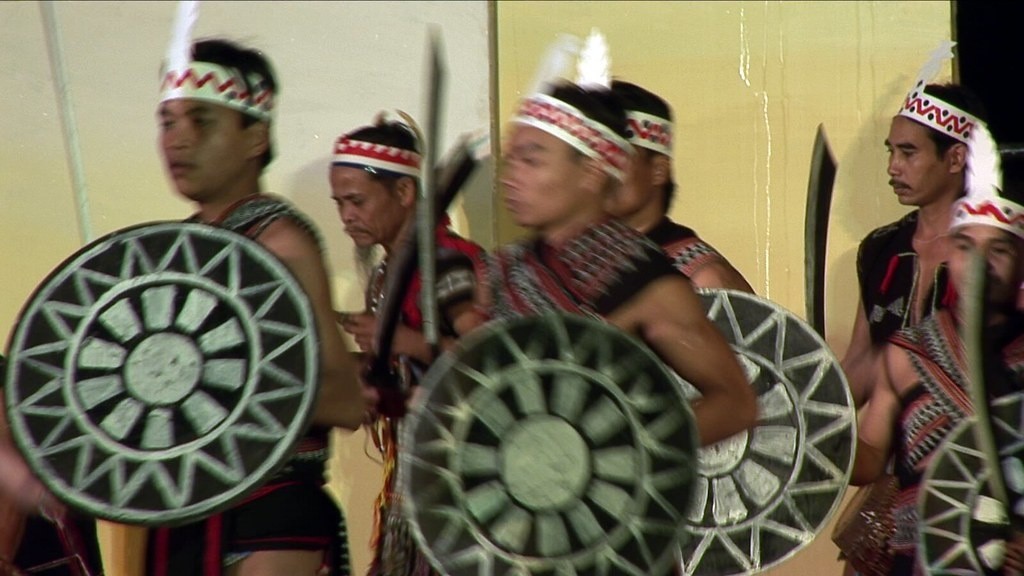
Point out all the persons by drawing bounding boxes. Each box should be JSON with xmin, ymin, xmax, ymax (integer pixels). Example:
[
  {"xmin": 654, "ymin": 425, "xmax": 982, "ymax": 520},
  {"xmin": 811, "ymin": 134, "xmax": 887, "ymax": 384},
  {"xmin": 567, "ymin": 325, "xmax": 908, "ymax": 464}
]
[
  {"xmin": 328, "ymin": 79, "xmax": 760, "ymax": 576},
  {"xmin": 142, "ymin": 38, "xmax": 365, "ymax": 576},
  {"xmin": 837, "ymin": 82, "xmax": 1024, "ymax": 576},
  {"xmin": 0, "ymin": 354, "xmax": 96, "ymax": 576}
]
